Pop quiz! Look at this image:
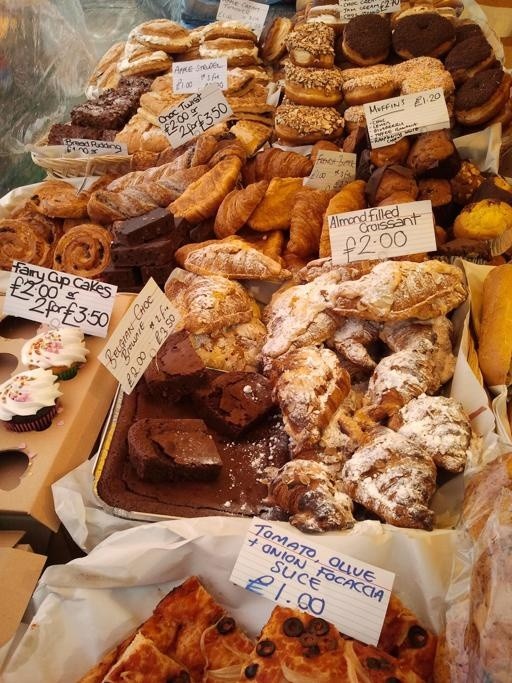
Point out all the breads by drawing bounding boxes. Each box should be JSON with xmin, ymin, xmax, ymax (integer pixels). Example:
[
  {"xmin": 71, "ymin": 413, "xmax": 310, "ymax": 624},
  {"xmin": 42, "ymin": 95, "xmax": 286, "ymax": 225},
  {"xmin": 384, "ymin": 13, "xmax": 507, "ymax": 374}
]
[{"xmin": 0, "ymin": 0, "xmax": 512, "ymax": 683}]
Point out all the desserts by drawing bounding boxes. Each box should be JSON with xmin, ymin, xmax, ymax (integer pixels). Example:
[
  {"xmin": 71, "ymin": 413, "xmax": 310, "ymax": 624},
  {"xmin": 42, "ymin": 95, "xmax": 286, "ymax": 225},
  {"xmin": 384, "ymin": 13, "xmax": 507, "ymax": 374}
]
[
  {"xmin": 1, "ymin": 367, "xmax": 65, "ymax": 433},
  {"xmin": 21, "ymin": 326, "xmax": 90, "ymax": 381}
]
